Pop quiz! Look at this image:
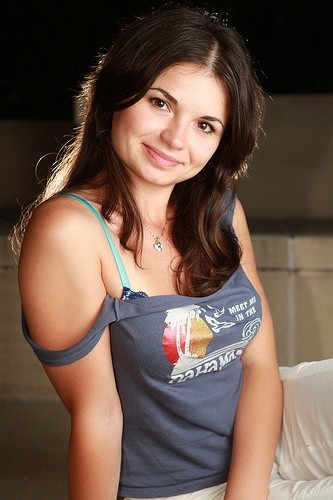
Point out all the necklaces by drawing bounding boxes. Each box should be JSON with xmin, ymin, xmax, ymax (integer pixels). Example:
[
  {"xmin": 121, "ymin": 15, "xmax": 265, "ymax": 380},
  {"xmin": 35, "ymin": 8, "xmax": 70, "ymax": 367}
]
[{"xmin": 139, "ymin": 214, "xmax": 171, "ymax": 253}]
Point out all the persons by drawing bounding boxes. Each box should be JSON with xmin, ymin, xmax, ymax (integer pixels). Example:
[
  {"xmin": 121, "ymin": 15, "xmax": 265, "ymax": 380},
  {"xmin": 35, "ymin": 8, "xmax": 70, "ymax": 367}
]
[{"xmin": 12, "ymin": 7, "xmax": 333, "ymax": 500}]
